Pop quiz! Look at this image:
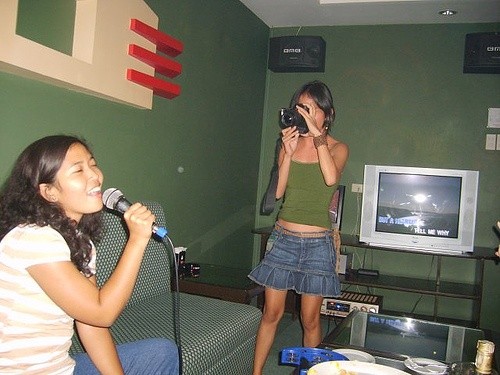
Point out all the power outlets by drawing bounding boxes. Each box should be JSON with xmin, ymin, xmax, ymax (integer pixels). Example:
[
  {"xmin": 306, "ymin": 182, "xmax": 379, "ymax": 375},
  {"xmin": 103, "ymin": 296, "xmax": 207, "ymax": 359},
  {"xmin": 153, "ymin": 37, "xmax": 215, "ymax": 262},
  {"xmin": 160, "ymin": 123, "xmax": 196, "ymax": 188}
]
[{"xmin": 351, "ymin": 183, "xmax": 363, "ymax": 193}]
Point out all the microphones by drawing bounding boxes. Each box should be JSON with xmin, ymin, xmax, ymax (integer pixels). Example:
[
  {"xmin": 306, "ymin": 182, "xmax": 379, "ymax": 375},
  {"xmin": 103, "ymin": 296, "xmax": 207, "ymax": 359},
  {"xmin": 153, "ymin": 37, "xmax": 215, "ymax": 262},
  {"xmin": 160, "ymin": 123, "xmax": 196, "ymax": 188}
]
[{"xmin": 102, "ymin": 188, "xmax": 169, "ymax": 238}]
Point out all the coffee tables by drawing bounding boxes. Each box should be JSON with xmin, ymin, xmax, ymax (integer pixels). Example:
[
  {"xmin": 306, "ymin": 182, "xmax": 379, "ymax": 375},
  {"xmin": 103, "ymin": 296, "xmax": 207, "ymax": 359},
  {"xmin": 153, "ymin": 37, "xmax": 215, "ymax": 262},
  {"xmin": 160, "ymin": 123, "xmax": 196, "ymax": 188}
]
[{"xmin": 292, "ymin": 309, "xmax": 486, "ymax": 375}]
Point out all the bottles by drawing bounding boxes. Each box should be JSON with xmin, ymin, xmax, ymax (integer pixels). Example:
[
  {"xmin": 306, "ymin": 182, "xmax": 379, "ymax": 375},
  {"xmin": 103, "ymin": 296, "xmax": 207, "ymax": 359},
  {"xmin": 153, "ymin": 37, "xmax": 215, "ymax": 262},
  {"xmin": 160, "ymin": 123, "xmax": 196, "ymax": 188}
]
[{"xmin": 474, "ymin": 339, "xmax": 495, "ymax": 375}]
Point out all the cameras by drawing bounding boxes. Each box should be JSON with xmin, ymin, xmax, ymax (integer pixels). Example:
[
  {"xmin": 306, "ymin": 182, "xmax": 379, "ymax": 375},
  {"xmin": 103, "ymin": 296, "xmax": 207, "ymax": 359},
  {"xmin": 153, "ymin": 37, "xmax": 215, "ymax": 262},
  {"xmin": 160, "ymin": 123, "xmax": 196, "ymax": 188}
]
[{"xmin": 279, "ymin": 103, "xmax": 311, "ymax": 134}]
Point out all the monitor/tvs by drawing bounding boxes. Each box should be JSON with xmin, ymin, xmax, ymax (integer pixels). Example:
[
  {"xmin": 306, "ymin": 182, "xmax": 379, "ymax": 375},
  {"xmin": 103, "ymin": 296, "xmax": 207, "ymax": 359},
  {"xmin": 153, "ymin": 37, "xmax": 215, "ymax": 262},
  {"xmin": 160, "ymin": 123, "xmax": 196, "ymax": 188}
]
[
  {"xmin": 350, "ymin": 311, "xmax": 465, "ymax": 362},
  {"xmin": 357, "ymin": 164, "xmax": 479, "ymax": 257}
]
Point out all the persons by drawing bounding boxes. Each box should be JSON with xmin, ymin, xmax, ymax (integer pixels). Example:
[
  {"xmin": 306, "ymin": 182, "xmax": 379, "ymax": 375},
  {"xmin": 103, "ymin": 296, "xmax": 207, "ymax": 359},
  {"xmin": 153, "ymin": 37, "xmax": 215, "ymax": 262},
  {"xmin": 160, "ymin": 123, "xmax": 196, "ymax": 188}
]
[
  {"xmin": 0, "ymin": 135, "xmax": 179, "ymax": 375},
  {"xmin": 247, "ymin": 80, "xmax": 348, "ymax": 375}
]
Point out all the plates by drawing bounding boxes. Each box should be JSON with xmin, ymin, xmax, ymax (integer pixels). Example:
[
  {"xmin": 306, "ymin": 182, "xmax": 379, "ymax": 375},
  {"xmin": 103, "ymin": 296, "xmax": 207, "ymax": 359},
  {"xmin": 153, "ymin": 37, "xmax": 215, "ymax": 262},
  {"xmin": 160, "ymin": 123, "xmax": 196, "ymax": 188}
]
[
  {"xmin": 332, "ymin": 349, "xmax": 376, "ymax": 364},
  {"xmin": 307, "ymin": 361, "xmax": 411, "ymax": 375},
  {"xmin": 403, "ymin": 357, "xmax": 448, "ymax": 375}
]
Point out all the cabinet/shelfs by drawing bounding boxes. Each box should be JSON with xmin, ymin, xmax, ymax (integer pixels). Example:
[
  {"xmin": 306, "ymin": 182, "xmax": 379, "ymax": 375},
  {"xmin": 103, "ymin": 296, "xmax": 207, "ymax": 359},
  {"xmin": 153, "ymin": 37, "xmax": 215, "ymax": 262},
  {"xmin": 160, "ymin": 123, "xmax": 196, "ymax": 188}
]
[{"xmin": 251, "ymin": 210, "xmax": 500, "ymax": 350}]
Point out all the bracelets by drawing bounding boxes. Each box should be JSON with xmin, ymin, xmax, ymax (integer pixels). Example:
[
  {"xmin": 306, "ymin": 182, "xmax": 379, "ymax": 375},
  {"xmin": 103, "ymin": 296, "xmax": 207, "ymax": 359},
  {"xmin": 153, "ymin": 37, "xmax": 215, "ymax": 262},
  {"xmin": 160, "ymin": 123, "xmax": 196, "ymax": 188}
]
[{"xmin": 313, "ymin": 135, "xmax": 328, "ymax": 149}]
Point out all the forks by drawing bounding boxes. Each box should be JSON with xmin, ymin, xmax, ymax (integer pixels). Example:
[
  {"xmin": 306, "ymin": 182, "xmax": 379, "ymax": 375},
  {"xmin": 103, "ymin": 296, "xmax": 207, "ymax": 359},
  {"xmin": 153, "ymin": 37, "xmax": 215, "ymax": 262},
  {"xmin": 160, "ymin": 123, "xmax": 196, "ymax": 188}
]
[{"xmin": 407, "ymin": 356, "xmax": 450, "ymax": 368}]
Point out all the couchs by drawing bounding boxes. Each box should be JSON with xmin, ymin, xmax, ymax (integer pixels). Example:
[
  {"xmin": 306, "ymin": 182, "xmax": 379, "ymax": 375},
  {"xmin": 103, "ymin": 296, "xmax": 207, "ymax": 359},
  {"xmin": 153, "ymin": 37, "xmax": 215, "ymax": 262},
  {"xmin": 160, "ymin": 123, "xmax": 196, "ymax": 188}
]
[{"xmin": 69, "ymin": 202, "xmax": 262, "ymax": 375}]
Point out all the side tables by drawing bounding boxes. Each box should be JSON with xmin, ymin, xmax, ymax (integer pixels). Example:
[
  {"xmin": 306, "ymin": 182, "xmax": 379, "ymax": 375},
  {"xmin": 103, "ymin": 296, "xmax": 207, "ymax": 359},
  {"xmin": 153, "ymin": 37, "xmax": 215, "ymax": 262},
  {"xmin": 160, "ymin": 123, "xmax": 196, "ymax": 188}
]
[{"xmin": 173, "ymin": 263, "xmax": 264, "ymax": 312}]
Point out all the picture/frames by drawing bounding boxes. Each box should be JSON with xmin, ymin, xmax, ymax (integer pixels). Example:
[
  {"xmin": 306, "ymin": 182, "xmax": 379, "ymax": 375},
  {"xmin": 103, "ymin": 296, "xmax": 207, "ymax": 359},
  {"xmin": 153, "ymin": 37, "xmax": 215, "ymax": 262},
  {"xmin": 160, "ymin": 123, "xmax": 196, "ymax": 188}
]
[{"xmin": 328, "ymin": 185, "xmax": 345, "ymax": 231}]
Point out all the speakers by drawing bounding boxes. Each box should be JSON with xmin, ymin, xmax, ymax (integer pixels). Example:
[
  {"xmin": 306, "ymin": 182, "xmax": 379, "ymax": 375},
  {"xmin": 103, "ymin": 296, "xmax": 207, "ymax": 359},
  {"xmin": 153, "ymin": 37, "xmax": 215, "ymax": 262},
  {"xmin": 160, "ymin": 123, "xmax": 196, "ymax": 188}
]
[
  {"xmin": 463, "ymin": 31, "xmax": 500, "ymax": 74},
  {"xmin": 268, "ymin": 34, "xmax": 326, "ymax": 73}
]
